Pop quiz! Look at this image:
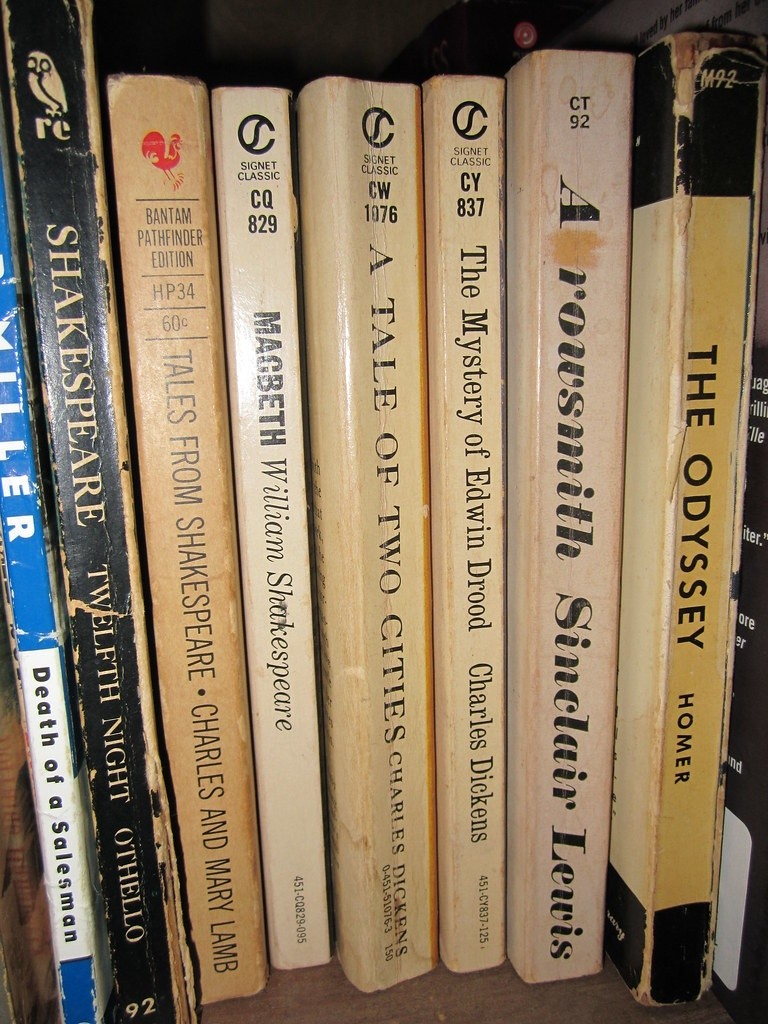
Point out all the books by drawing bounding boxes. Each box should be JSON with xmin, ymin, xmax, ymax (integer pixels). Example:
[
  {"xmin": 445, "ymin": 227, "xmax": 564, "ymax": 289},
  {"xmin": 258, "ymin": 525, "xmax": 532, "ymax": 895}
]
[{"xmin": 0, "ymin": 0, "xmax": 768, "ymax": 1024}]
